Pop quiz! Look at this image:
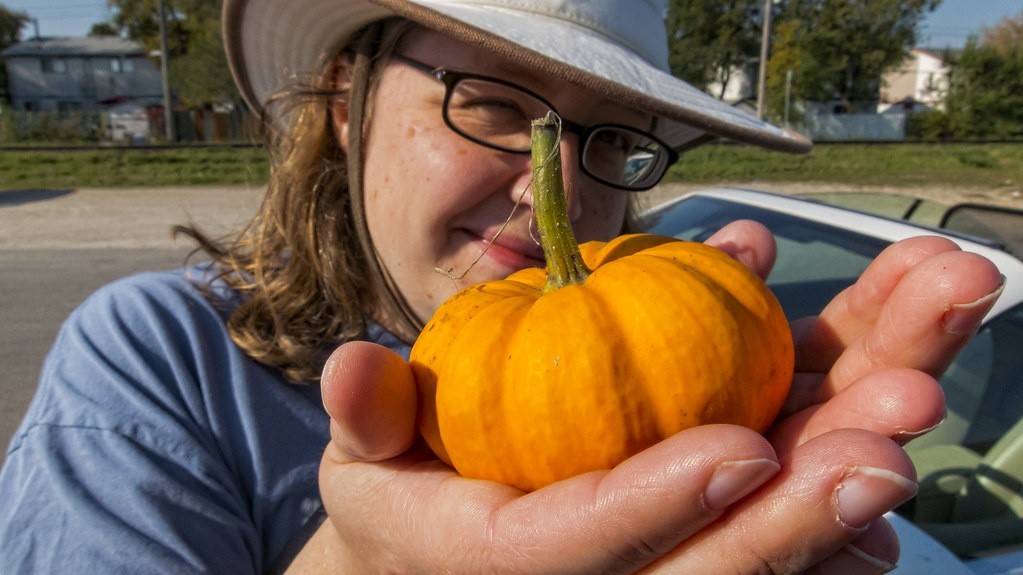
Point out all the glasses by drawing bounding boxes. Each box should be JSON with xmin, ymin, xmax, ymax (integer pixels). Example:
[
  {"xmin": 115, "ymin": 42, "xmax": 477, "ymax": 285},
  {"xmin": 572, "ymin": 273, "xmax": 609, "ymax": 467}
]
[{"xmin": 393, "ymin": 53, "xmax": 682, "ymax": 192}]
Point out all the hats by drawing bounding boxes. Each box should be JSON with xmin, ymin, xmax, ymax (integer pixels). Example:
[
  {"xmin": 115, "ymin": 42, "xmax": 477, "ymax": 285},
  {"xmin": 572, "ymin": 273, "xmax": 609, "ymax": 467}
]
[{"xmin": 219, "ymin": 0, "xmax": 818, "ymax": 156}]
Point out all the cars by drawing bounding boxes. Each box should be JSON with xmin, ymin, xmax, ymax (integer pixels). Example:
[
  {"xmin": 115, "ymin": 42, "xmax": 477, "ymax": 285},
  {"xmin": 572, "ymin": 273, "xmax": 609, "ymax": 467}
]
[{"xmin": 623, "ymin": 187, "xmax": 1023, "ymax": 575}]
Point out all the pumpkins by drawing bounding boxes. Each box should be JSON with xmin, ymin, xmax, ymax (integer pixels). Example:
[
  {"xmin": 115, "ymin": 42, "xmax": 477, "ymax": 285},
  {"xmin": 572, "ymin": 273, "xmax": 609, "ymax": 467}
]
[{"xmin": 405, "ymin": 108, "xmax": 796, "ymax": 497}]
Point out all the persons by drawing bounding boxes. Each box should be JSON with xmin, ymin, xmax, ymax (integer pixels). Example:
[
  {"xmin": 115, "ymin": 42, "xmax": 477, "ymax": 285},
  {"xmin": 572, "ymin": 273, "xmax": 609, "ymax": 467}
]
[{"xmin": 1, "ymin": 0, "xmax": 1009, "ymax": 575}]
